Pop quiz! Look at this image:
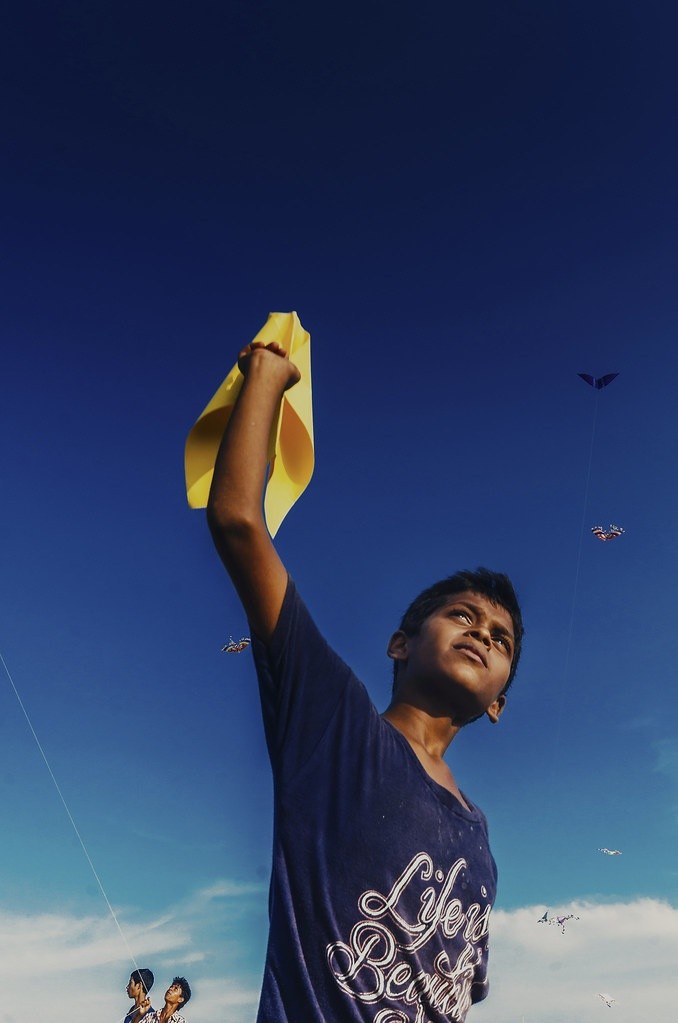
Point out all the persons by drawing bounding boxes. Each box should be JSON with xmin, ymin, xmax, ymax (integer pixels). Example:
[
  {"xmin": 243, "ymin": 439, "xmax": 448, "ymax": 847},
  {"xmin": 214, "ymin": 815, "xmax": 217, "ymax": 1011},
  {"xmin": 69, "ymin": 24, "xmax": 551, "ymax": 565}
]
[
  {"xmin": 204, "ymin": 309, "xmax": 525, "ymax": 1023},
  {"xmin": 131, "ymin": 976, "xmax": 193, "ymax": 1022},
  {"xmin": 122, "ymin": 968, "xmax": 158, "ymax": 1023}
]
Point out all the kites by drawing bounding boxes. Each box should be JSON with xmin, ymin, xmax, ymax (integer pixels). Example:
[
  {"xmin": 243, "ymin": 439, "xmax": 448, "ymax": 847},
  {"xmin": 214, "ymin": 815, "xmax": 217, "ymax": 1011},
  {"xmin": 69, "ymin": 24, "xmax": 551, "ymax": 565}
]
[
  {"xmin": 578, "ymin": 369, "xmax": 621, "ymax": 388},
  {"xmin": 590, "ymin": 523, "xmax": 626, "ymax": 541},
  {"xmin": 597, "ymin": 848, "xmax": 622, "ymax": 856},
  {"xmin": 537, "ymin": 910, "xmax": 579, "ymax": 934},
  {"xmin": 600, "ymin": 992, "xmax": 615, "ymax": 1008},
  {"xmin": 222, "ymin": 636, "xmax": 251, "ymax": 654}
]
